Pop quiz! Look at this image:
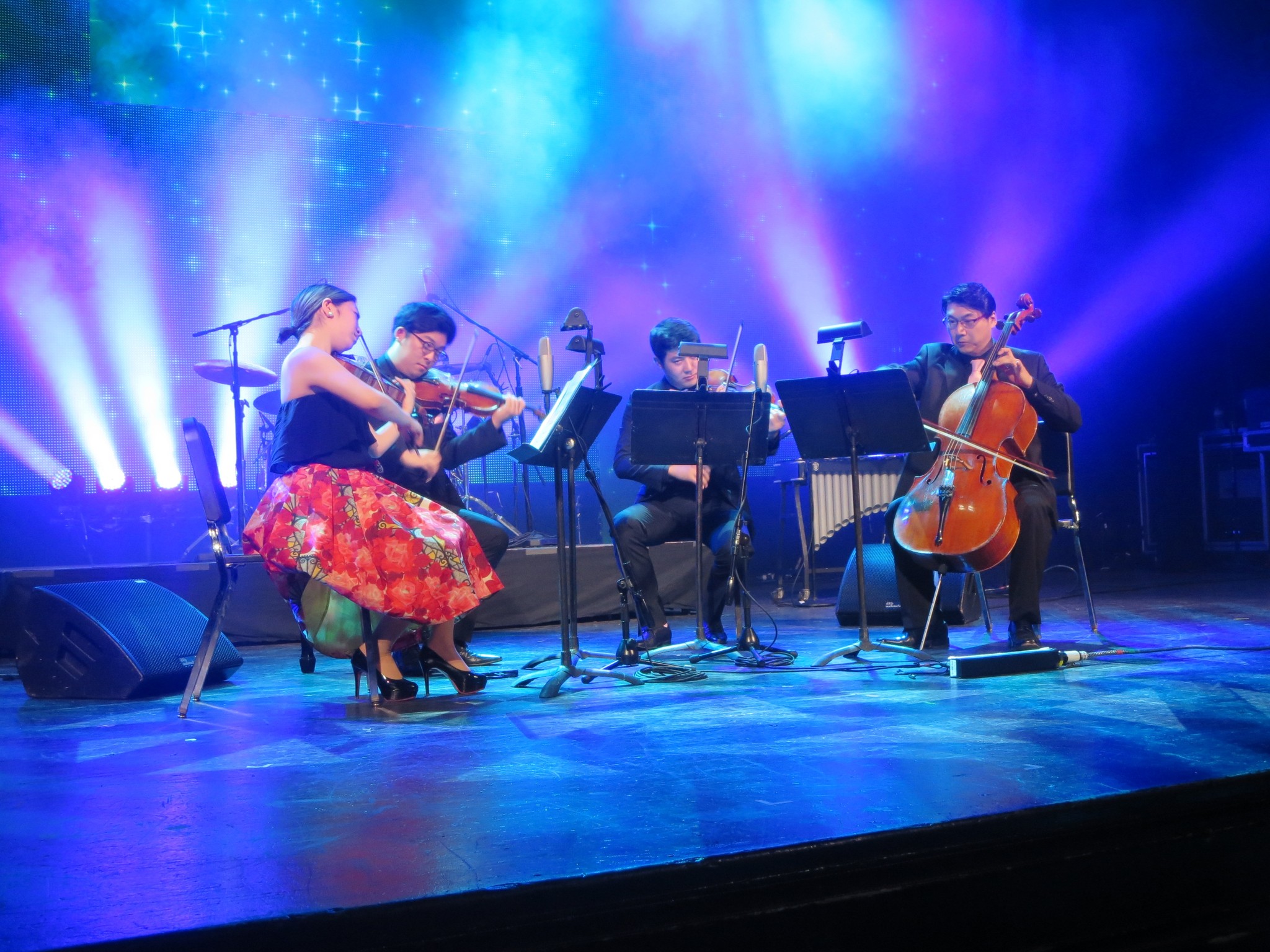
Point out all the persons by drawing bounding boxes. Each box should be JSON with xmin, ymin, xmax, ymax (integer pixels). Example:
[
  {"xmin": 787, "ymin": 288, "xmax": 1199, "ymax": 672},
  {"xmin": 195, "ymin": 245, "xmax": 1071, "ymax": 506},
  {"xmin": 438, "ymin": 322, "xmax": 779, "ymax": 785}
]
[
  {"xmin": 875, "ymin": 282, "xmax": 1083, "ymax": 652},
  {"xmin": 612, "ymin": 318, "xmax": 786, "ymax": 652},
  {"xmin": 240, "ymin": 282, "xmax": 488, "ymax": 701},
  {"xmin": 360, "ymin": 301, "xmax": 526, "ymax": 677}
]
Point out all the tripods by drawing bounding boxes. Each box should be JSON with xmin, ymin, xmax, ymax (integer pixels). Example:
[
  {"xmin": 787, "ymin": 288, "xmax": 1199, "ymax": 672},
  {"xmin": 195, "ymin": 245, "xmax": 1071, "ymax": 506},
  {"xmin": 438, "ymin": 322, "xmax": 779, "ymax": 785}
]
[{"xmin": 512, "ymin": 334, "xmax": 935, "ymax": 699}]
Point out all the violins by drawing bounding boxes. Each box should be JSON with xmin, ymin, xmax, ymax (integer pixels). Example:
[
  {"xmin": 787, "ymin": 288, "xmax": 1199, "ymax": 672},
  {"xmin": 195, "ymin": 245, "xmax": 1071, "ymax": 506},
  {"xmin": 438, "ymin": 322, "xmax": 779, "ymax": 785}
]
[
  {"xmin": 708, "ymin": 368, "xmax": 785, "ymax": 413},
  {"xmin": 411, "ymin": 367, "xmax": 547, "ymax": 422},
  {"xmin": 332, "ymin": 351, "xmax": 435, "ymax": 431}
]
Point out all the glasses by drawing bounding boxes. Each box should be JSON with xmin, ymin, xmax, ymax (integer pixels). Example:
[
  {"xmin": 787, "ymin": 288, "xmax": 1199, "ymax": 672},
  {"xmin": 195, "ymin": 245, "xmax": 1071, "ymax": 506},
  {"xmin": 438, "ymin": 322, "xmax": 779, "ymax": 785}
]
[
  {"xmin": 942, "ymin": 314, "xmax": 985, "ymax": 330},
  {"xmin": 409, "ymin": 330, "xmax": 448, "ymax": 362}
]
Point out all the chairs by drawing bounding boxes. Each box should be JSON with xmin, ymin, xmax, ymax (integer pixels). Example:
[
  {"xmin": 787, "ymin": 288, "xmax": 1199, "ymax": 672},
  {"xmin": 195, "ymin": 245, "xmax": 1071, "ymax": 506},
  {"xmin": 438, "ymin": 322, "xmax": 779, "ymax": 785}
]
[
  {"xmin": 178, "ymin": 417, "xmax": 379, "ymax": 718},
  {"xmin": 972, "ymin": 381, "xmax": 1097, "ymax": 632}
]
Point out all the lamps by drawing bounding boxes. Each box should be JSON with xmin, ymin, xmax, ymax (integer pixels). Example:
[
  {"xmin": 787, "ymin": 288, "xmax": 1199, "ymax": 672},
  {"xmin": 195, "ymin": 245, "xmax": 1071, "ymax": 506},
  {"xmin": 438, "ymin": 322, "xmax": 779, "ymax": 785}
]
[{"xmin": 49, "ymin": 469, "xmax": 86, "ymax": 495}]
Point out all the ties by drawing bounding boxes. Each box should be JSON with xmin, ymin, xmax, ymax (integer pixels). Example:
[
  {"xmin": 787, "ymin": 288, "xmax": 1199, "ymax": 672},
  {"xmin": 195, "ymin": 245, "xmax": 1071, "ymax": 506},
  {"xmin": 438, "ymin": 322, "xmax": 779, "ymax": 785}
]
[{"xmin": 968, "ymin": 359, "xmax": 985, "ymax": 384}]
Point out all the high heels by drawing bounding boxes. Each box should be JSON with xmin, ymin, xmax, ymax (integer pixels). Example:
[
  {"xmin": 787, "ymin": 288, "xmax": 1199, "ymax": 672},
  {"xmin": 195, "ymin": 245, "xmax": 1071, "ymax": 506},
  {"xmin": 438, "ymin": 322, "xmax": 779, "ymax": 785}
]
[
  {"xmin": 350, "ymin": 648, "xmax": 418, "ymax": 701},
  {"xmin": 418, "ymin": 642, "xmax": 487, "ymax": 696}
]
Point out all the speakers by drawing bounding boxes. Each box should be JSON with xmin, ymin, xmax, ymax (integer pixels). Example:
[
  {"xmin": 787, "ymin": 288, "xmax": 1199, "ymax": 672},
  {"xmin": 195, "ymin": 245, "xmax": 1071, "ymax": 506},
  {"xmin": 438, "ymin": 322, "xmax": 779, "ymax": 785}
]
[
  {"xmin": 835, "ymin": 542, "xmax": 983, "ymax": 626},
  {"xmin": 16, "ymin": 576, "xmax": 242, "ymax": 700}
]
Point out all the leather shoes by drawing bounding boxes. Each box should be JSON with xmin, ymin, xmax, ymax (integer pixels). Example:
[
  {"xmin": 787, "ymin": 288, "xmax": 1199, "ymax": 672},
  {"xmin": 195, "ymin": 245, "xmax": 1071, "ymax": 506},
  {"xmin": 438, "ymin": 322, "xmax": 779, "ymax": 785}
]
[
  {"xmin": 876, "ymin": 621, "xmax": 950, "ymax": 649},
  {"xmin": 454, "ymin": 643, "xmax": 503, "ymax": 667},
  {"xmin": 702, "ymin": 623, "xmax": 727, "ymax": 644},
  {"xmin": 1008, "ymin": 622, "xmax": 1042, "ymax": 651},
  {"xmin": 392, "ymin": 650, "xmax": 431, "ymax": 679},
  {"xmin": 635, "ymin": 625, "xmax": 672, "ymax": 649}
]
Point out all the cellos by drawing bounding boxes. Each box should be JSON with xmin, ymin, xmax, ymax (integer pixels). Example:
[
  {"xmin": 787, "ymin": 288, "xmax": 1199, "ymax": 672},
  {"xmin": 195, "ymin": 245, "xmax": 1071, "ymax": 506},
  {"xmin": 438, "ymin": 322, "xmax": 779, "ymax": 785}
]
[{"xmin": 893, "ymin": 292, "xmax": 1042, "ymax": 651}]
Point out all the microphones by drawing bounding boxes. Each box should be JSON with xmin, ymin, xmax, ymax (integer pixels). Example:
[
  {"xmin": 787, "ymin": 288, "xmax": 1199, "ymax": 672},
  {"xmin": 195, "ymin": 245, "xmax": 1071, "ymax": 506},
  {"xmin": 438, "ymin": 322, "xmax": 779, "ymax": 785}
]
[
  {"xmin": 536, "ymin": 335, "xmax": 555, "ymax": 394},
  {"xmin": 422, "ymin": 274, "xmax": 435, "ymax": 306},
  {"xmin": 754, "ymin": 343, "xmax": 768, "ymax": 392}
]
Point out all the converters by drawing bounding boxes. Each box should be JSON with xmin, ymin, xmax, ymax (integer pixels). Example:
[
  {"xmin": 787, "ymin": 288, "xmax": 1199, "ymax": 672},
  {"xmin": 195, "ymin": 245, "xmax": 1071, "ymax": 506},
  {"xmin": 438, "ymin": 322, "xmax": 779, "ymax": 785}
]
[{"xmin": 951, "ymin": 645, "xmax": 1057, "ymax": 677}]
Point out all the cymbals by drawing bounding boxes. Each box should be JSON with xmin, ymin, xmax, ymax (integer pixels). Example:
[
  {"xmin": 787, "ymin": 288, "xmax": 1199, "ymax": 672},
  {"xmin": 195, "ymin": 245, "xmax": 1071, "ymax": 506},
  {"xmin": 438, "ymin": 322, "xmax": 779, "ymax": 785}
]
[
  {"xmin": 432, "ymin": 363, "xmax": 479, "ymax": 375},
  {"xmin": 253, "ymin": 389, "xmax": 282, "ymax": 416},
  {"xmin": 193, "ymin": 359, "xmax": 277, "ymax": 387}
]
